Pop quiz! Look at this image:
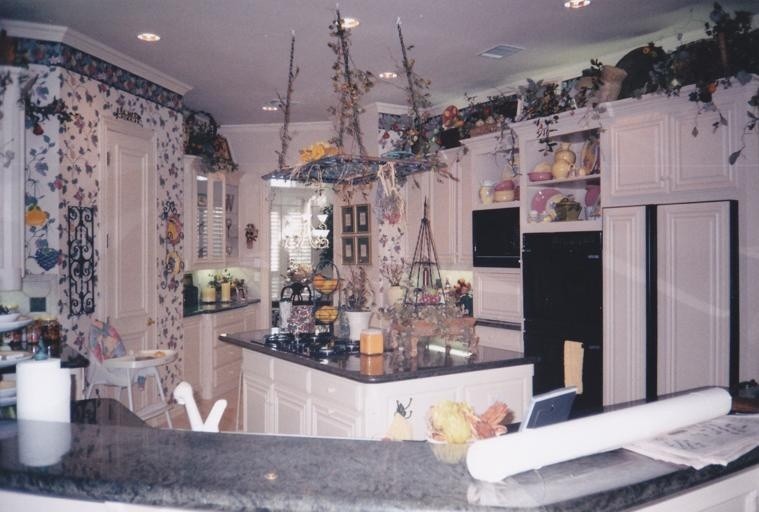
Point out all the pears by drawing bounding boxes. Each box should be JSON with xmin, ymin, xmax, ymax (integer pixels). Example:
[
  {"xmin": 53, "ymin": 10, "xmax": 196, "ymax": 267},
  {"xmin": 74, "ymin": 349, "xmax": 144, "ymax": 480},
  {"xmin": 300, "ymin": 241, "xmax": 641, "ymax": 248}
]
[{"xmin": 315, "ymin": 306, "xmax": 337, "ymax": 320}]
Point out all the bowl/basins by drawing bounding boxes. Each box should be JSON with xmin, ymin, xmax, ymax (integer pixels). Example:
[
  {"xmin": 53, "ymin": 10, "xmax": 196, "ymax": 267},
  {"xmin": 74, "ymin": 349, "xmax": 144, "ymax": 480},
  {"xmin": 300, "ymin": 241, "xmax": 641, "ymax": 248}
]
[
  {"xmin": 494, "ymin": 191, "xmax": 514, "ymax": 202},
  {"xmin": 527, "ymin": 173, "xmax": 552, "ymax": 181},
  {"xmin": 496, "ymin": 179, "xmax": 514, "ymax": 191}
]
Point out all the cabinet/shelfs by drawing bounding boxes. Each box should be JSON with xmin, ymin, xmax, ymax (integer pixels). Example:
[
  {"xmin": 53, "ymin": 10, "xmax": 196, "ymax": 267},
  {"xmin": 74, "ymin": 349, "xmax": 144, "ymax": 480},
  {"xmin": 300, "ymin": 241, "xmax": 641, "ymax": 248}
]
[
  {"xmin": 180, "ymin": 149, "xmax": 245, "ymax": 269},
  {"xmin": 471, "ymin": 268, "xmax": 522, "ymax": 327},
  {"xmin": 475, "ymin": 323, "xmax": 525, "ymax": 352},
  {"xmin": 331, "ymin": 179, "xmax": 389, "ymax": 329},
  {"xmin": 601, "ymin": 202, "xmax": 759, "ymax": 406},
  {"xmin": 241, "ymin": 347, "xmax": 311, "ymax": 438},
  {"xmin": 199, "ymin": 307, "xmax": 245, "ymax": 400},
  {"xmin": 459, "ymin": 131, "xmax": 519, "ymax": 268},
  {"xmin": 510, "ymin": 107, "xmax": 603, "ymax": 232},
  {"xmin": 601, "ymin": 73, "xmax": 759, "ymax": 205},
  {"xmin": 183, "ymin": 314, "xmax": 201, "ymax": 394},
  {"xmin": 399, "ymin": 149, "xmax": 457, "ymax": 271},
  {"xmin": 376, "ymin": 365, "xmax": 534, "ymax": 439},
  {"xmin": 244, "ymin": 301, "xmax": 260, "ymax": 332},
  {"xmin": 309, "ymin": 366, "xmax": 363, "ymax": 440}
]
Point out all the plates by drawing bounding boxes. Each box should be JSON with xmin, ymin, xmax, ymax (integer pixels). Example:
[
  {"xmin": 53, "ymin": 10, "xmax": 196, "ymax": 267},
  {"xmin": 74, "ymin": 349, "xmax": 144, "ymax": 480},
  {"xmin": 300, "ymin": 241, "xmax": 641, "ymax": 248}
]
[
  {"xmin": 531, "ymin": 188, "xmax": 565, "ymax": 220},
  {"xmin": 581, "ymin": 135, "xmax": 598, "ymax": 175},
  {"xmin": 0, "ymin": 311, "xmax": 38, "ymax": 407}
]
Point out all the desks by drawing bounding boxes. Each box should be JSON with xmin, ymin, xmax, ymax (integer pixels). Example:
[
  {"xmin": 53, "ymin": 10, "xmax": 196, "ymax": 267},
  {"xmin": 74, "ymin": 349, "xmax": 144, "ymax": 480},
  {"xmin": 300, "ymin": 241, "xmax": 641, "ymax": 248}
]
[
  {"xmin": 3, "ymin": 337, "xmax": 90, "ymax": 402},
  {"xmin": 104, "ymin": 347, "xmax": 179, "ymax": 370}
]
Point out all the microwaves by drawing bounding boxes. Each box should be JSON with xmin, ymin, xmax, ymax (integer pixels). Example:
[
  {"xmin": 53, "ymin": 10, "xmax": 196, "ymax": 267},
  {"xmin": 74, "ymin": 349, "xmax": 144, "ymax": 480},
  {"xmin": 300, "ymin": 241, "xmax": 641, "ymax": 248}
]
[{"xmin": 471, "ymin": 206, "xmax": 522, "ymax": 270}]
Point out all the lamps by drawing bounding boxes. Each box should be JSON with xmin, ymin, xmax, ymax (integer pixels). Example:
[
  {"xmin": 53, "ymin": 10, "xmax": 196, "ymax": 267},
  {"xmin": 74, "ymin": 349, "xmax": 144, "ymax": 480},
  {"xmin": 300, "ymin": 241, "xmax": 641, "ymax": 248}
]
[{"xmin": 277, "ymin": 206, "xmax": 331, "ymax": 255}]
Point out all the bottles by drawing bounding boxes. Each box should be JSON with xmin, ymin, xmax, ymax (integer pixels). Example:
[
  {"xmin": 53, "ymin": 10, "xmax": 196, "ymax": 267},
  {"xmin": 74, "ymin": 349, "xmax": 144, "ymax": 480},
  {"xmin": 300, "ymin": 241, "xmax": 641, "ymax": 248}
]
[
  {"xmin": 359, "ymin": 354, "xmax": 385, "ymax": 378},
  {"xmin": 554, "ymin": 142, "xmax": 576, "ymax": 164},
  {"xmin": 556, "ymin": 198, "xmax": 577, "ymax": 221},
  {"xmin": 552, "ymin": 160, "xmax": 570, "ymax": 180},
  {"xmin": 359, "ymin": 327, "xmax": 385, "ymax": 355}
]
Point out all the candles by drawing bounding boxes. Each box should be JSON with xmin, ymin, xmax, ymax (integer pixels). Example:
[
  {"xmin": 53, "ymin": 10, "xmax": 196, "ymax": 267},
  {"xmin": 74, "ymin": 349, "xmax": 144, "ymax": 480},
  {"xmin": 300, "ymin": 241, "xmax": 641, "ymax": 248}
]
[
  {"xmin": 221, "ymin": 283, "xmax": 231, "ymax": 300},
  {"xmin": 201, "ymin": 287, "xmax": 215, "ymax": 302}
]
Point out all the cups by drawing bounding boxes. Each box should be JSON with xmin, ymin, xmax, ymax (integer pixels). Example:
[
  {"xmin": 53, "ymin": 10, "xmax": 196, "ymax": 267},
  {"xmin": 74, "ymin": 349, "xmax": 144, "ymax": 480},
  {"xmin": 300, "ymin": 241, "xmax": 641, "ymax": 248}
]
[
  {"xmin": 583, "ymin": 184, "xmax": 601, "ymax": 220},
  {"xmin": 200, "ymin": 287, "xmax": 216, "ymax": 304},
  {"xmin": 221, "ymin": 282, "xmax": 230, "ymax": 301}
]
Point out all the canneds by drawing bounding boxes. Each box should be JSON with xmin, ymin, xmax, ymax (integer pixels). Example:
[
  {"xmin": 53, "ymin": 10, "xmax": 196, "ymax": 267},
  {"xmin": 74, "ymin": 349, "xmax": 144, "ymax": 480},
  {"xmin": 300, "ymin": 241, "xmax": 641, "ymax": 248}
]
[{"xmin": 7, "ymin": 315, "xmax": 60, "ymax": 344}]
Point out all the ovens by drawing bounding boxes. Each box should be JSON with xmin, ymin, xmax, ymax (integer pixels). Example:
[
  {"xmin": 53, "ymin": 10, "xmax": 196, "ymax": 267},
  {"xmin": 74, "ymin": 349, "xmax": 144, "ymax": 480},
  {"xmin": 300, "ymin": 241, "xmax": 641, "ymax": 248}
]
[{"xmin": 523, "ymin": 230, "xmax": 603, "ymax": 420}]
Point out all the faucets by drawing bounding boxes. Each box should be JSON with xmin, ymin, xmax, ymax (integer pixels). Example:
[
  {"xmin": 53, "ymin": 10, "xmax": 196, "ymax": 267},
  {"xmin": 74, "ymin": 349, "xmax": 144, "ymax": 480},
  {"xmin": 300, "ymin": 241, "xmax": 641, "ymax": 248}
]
[{"xmin": 368, "ymin": 311, "xmax": 380, "ymax": 329}]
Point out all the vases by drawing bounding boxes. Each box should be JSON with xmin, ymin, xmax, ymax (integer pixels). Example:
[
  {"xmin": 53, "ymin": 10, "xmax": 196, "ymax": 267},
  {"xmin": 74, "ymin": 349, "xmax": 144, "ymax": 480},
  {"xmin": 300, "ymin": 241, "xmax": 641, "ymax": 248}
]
[
  {"xmin": 387, "ymin": 284, "xmax": 404, "ymax": 308},
  {"xmin": 345, "ymin": 310, "xmax": 372, "ymax": 342},
  {"xmin": 555, "ymin": 141, "xmax": 577, "ymax": 166}
]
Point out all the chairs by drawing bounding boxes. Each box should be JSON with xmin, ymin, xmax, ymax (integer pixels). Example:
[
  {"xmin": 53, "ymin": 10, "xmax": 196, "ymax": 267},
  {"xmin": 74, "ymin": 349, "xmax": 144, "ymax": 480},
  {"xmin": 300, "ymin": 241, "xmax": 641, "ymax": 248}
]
[{"xmin": 81, "ymin": 321, "xmax": 173, "ymax": 429}]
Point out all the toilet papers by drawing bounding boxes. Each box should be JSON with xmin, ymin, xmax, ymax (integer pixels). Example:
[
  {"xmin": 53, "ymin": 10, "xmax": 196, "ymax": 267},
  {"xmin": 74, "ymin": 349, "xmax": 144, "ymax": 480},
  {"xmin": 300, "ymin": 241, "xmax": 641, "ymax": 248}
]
[
  {"xmin": 15, "ymin": 358, "xmax": 71, "ymax": 423},
  {"xmin": 16, "ymin": 419, "xmax": 72, "ymax": 467},
  {"xmin": 465, "ymin": 387, "xmax": 733, "ymax": 484}
]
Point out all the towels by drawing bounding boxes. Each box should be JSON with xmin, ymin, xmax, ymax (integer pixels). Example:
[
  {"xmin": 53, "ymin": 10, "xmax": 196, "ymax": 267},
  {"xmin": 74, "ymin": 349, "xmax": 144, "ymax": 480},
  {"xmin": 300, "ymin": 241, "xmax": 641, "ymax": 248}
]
[{"xmin": 563, "ymin": 339, "xmax": 585, "ymax": 395}]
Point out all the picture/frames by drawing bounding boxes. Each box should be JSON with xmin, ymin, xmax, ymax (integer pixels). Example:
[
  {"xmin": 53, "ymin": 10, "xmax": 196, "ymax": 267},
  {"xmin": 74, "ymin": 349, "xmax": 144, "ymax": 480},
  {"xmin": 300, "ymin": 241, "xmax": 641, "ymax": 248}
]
[
  {"xmin": 340, "ymin": 203, "xmax": 355, "ymax": 235},
  {"xmin": 357, "ymin": 235, "xmax": 373, "ymax": 266},
  {"xmin": 355, "ymin": 203, "xmax": 372, "ymax": 234},
  {"xmin": 340, "ymin": 236, "xmax": 356, "ymax": 265}
]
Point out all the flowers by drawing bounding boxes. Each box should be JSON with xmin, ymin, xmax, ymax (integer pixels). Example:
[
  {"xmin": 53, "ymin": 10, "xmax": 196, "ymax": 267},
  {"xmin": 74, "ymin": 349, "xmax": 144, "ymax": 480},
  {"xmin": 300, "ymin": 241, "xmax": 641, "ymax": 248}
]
[{"xmin": 24, "ymin": 98, "xmax": 68, "ymax": 134}]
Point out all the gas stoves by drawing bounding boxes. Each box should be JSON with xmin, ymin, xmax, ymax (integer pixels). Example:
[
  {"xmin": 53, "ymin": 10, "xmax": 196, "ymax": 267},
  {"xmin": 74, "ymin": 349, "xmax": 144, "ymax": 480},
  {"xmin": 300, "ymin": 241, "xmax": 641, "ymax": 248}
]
[{"xmin": 247, "ymin": 333, "xmax": 358, "ymax": 362}]
